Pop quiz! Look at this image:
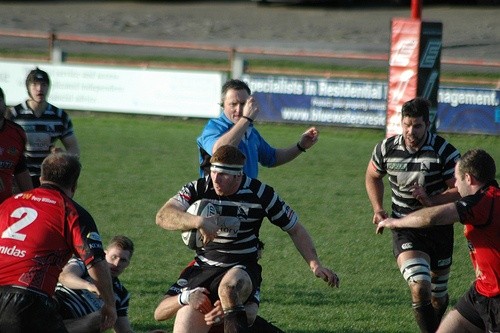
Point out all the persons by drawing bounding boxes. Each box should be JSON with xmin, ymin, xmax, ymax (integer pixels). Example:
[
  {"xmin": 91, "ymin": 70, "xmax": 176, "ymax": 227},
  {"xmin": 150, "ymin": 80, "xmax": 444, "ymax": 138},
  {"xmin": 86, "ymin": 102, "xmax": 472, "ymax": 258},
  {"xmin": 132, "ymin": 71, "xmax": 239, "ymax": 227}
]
[
  {"xmin": 377, "ymin": 149, "xmax": 500, "ymax": 333},
  {"xmin": 365, "ymin": 98, "xmax": 461, "ymax": 333},
  {"xmin": 154, "ymin": 146, "xmax": 339, "ymax": 333},
  {"xmin": 197, "ymin": 79, "xmax": 320, "ymax": 184},
  {"xmin": 0, "ymin": 69, "xmax": 135, "ymax": 333}
]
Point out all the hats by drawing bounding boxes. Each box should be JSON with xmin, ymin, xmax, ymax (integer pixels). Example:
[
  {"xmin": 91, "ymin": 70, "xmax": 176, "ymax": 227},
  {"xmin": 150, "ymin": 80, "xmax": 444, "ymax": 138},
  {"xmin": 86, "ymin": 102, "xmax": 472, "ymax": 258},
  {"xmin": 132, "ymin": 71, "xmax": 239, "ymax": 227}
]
[{"xmin": 26, "ymin": 68, "xmax": 51, "ymax": 85}]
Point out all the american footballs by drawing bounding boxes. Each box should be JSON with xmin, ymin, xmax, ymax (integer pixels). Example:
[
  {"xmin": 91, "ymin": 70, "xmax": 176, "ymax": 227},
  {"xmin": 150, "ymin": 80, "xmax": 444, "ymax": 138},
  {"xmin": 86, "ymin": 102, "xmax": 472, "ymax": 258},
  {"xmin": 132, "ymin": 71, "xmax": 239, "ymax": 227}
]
[{"xmin": 182, "ymin": 200, "xmax": 219, "ymax": 250}]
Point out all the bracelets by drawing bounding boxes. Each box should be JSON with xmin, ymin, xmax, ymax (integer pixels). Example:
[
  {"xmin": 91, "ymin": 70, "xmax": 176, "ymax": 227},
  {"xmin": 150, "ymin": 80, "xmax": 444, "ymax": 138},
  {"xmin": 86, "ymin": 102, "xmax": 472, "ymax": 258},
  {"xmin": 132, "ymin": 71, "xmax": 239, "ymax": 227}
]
[
  {"xmin": 242, "ymin": 116, "xmax": 254, "ymax": 123},
  {"xmin": 297, "ymin": 143, "xmax": 306, "ymax": 153}
]
